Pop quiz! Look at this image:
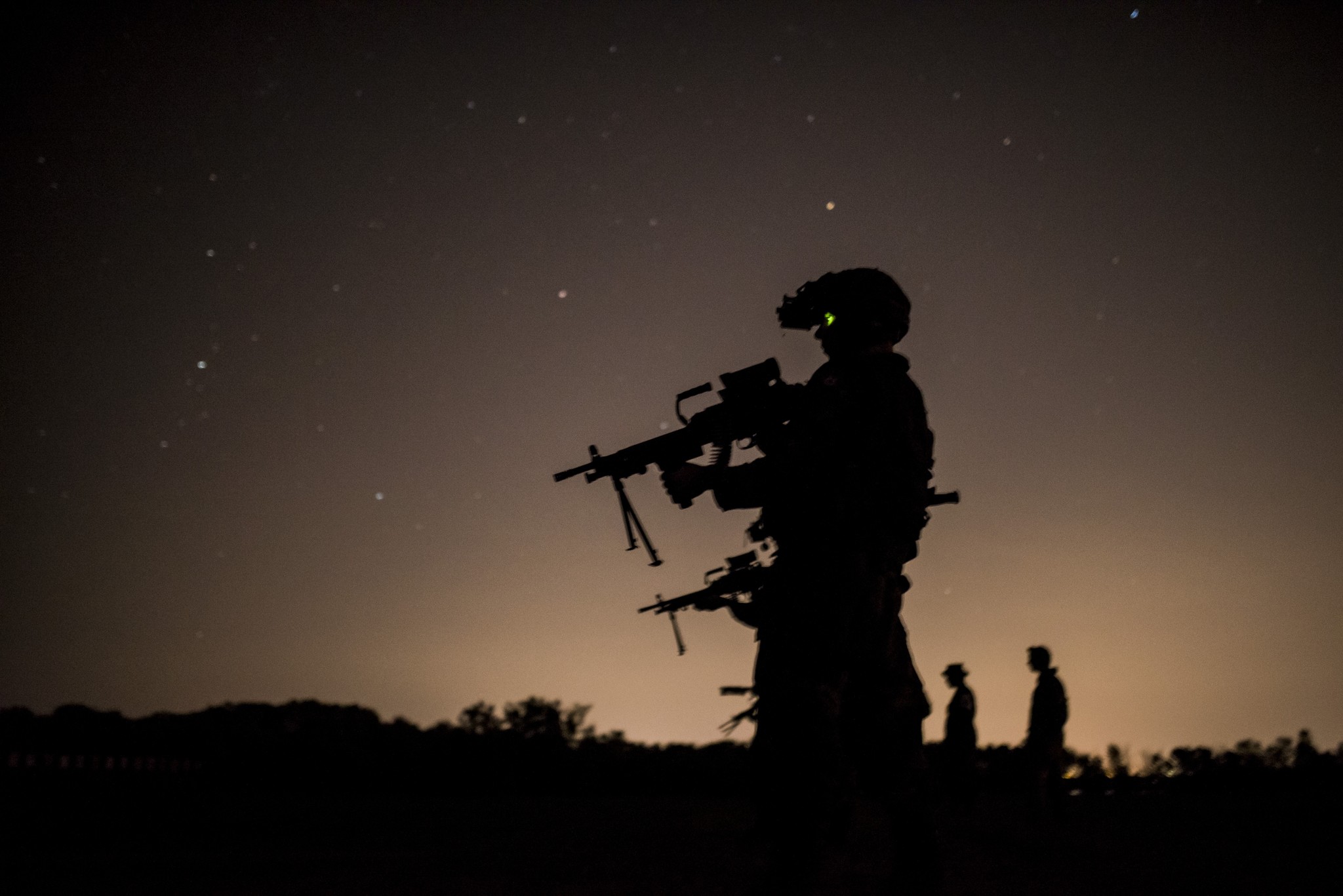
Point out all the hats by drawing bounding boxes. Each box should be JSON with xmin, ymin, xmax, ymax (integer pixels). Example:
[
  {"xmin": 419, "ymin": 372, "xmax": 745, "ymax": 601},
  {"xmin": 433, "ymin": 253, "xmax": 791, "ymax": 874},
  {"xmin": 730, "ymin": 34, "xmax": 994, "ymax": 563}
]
[{"xmin": 942, "ymin": 664, "xmax": 968, "ymax": 677}]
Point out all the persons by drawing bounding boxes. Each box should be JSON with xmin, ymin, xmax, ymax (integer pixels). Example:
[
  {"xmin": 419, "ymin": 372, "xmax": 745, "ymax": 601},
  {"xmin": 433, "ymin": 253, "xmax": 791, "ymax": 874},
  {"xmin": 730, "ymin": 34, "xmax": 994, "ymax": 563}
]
[
  {"xmin": 1024, "ymin": 642, "xmax": 1072, "ymax": 755},
  {"xmin": 938, "ymin": 663, "xmax": 981, "ymax": 748},
  {"xmin": 655, "ymin": 267, "xmax": 936, "ymax": 759}
]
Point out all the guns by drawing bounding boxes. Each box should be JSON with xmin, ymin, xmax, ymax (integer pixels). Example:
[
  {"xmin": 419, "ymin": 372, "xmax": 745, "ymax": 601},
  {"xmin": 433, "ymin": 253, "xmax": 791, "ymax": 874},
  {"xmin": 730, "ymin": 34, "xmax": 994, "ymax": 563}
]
[
  {"xmin": 717, "ymin": 682, "xmax": 757, "ymax": 739},
  {"xmin": 637, "ymin": 549, "xmax": 776, "ymax": 657},
  {"xmin": 551, "ymin": 355, "xmax": 797, "ymax": 570}
]
